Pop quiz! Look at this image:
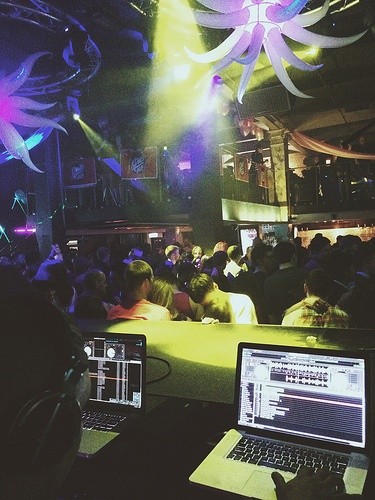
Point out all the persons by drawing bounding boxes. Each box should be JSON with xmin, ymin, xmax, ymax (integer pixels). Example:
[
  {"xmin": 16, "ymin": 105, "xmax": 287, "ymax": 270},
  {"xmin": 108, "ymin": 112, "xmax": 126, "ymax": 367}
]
[
  {"xmin": 288, "ymin": 134, "xmax": 375, "ymax": 211},
  {"xmin": 0, "ymin": 295, "xmax": 363, "ymax": 500},
  {"xmin": 248, "ymin": 144, "xmax": 269, "ymax": 204},
  {"xmin": 0, "ymin": 234, "xmax": 375, "ymax": 330}
]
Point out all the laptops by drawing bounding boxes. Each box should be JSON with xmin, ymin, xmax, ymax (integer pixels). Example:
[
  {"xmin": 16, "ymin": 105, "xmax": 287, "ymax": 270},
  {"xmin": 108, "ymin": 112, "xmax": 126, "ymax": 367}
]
[
  {"xmin": 63, "ymin": 331, "xmax": 147, "ymax": 461},
  {"xmin": 188, "ymin": 340, "xmax": 374, "ymax": 500}
]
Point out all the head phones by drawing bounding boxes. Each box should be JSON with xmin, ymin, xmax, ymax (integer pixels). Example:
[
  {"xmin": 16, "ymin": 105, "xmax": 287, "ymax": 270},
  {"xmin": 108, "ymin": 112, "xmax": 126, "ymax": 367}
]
[{"xmin": 1, "ymin": 310, "xmax": 84, "ymax": 485}]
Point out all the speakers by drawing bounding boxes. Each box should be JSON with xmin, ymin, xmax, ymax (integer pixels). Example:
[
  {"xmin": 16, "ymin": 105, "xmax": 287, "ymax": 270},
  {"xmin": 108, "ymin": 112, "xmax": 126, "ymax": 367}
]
[{"xmin": 233, "ymin": 83, "xmax": 292, "ymax": 121}]
